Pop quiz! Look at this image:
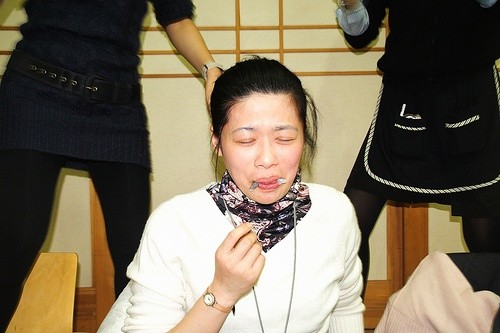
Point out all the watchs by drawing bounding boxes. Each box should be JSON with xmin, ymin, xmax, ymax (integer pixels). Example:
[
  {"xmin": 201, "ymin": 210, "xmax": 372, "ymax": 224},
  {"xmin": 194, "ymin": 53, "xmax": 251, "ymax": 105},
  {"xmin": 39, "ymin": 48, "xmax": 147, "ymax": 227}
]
[{"xmin": 204, "ymin": 287, "xmax": 231, "ymax": 314}]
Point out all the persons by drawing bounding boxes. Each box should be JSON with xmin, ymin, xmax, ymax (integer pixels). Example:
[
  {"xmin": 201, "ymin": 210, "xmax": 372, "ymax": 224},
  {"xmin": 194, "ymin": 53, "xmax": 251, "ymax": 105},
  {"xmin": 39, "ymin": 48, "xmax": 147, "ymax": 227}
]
[
  {"xmin": 335, "ymin": 0, "xmax": 500, "ymax": 295},
  {"xmin": 97, "ymin": 57, "xmax": 366, "ymax": 333},
  {"xmin": 0, "ymin": 0, "xmax": 222, "ymax": 333},
  {"xmin": 374, "ymin": 251, "xmax": 500, "ymax": 333}
]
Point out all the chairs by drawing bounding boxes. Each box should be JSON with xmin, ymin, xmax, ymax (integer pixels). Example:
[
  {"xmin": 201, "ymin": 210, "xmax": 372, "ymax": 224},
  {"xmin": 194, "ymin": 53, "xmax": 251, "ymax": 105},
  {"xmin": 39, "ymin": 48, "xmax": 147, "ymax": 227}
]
[{"xmin": 6, "ymin": 252, "xmax": 78, "ymax": 333}]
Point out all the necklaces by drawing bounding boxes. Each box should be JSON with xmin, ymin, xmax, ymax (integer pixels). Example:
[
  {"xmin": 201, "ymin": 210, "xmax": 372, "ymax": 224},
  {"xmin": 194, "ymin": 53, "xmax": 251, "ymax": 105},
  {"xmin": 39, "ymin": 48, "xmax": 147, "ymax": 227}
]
[{"xmin": 223, "ymin": 200, "xmax": 297, "ymax": 333}]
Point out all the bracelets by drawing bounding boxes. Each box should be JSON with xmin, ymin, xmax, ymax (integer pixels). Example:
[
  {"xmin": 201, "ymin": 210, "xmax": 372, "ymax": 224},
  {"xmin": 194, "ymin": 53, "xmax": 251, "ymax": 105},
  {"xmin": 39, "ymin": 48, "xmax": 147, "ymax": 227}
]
[{"xmin": 202, "ymin": 62, "xmax": 225, "ymax": 81}]
